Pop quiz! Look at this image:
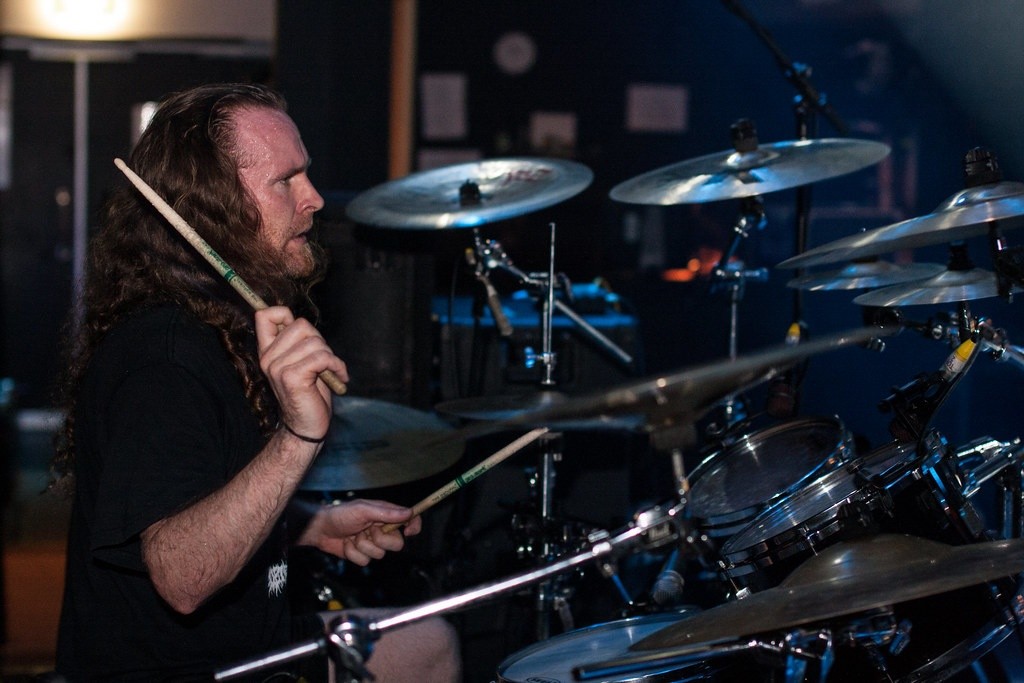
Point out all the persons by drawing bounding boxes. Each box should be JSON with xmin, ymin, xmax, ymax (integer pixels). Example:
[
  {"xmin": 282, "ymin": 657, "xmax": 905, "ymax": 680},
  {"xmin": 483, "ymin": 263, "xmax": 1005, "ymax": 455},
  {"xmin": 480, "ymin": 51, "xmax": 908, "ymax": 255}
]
[{"xmin": 42, "ymin": 80, "xmax": 467, "ymax": 683}]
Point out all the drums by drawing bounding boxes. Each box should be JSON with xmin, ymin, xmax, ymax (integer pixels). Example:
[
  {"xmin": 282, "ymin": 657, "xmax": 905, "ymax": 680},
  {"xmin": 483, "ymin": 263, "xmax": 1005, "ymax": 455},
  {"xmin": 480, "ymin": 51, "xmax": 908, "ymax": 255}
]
[
  {"xmin": 711, "ymin": 429, "xmax": 1024, "ymax": 683},
  {"xmin": 488, "ymin": 605, "xmax": 752, "ymax": 683},
  {"xmin": 675, "ymin": 412, "xmax": 860, "ymax": 564}
]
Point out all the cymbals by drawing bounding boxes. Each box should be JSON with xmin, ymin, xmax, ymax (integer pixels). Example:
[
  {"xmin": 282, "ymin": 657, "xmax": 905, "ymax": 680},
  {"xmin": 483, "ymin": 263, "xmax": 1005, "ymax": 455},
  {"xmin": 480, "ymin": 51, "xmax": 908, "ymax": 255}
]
[
  {"xmin": 627, "ymin": 531, "xmax": 1024, "ymax": 654},
  {"xmin": 295, "ymin": 391, "xmax": 462, "ymax": 492},
  {"xmin": 775, "ymin": 178, "xmax": 1024, "ymax": 273},
  {"xmin": 784, "ymin": 257, "xmax": 948, "ymax": 290},
  {"xmin": 609, "ymin": 136, "xmax": 892, "ymax": 207},
  {"xmin": 851, "ymin": 266, "xmax": 1024, "ymax": 306},
  {"xmin": 432, "ymin": 388, "xmax": 652, "ymax": 431},
  {"xmin": 345, "ymin": 153, "xmax": 595, "ymax": 232},
  {"xmin": 456, "ymin": 317, "xmax": 906, "ymax": 442}
]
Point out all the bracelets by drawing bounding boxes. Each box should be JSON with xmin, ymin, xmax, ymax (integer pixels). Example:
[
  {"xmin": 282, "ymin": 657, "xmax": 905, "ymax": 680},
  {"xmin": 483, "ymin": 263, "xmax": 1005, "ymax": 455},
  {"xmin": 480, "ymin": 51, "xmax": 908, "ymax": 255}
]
[{"xmin": 279, "ymin": 418, "xmax": 328, "ymax": 443}]
